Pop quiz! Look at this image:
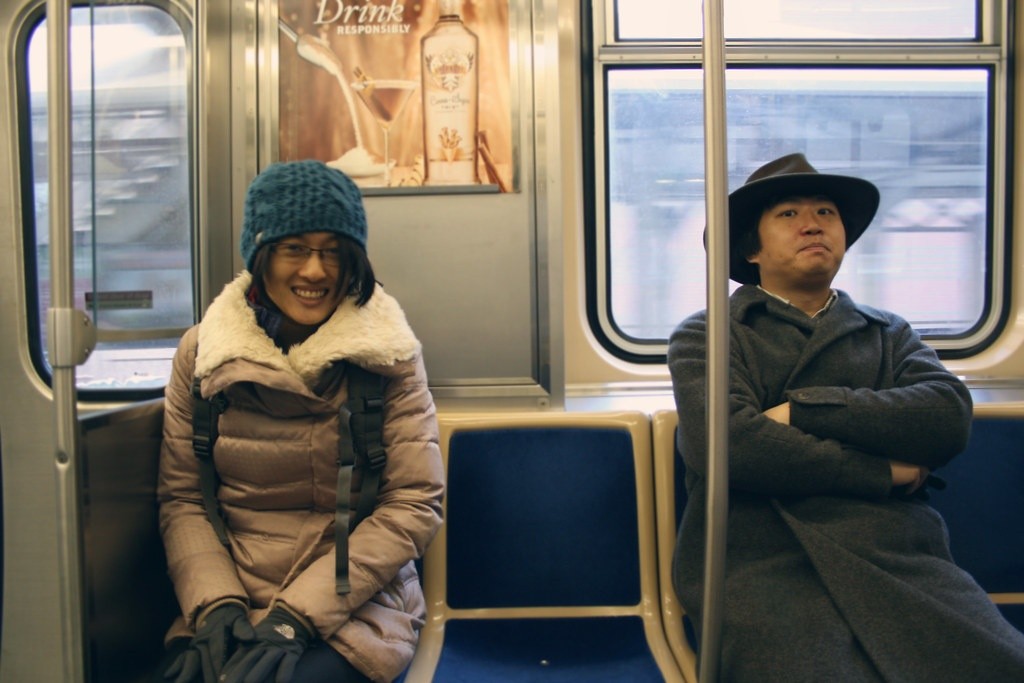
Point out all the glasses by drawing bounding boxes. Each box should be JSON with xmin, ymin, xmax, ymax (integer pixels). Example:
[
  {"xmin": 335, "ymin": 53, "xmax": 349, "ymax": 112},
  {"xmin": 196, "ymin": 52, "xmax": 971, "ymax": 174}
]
[{"xmin": 271, "ymin": 242, "xmax": 345, "ymax": 267}]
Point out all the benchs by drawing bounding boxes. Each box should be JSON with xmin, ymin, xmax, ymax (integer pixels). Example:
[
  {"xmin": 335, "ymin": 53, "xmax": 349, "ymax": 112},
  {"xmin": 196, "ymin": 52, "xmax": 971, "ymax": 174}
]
[{"xmin": 395, "ymin": 398, "xmax": 1024, "ymax": 683}]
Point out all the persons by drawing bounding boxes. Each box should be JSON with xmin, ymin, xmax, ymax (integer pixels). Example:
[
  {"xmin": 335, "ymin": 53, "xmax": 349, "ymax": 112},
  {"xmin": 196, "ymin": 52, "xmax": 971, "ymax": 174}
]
[
  {"xmin": 139, "ymin": 161, "xmax": 445, "ymax": 683},
  {"xmin": 668, "ymin": 152, "xmax": 1024, "ymax": 683}
]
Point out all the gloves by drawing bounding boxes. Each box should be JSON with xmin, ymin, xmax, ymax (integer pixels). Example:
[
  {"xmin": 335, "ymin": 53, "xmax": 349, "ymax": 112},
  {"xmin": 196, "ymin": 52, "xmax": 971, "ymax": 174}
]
[
  {"xmin": 219, "ymin": 604, "xmax": 311, "ymax": 683},
  {"xmin": 163, "ymin": 601, "xmax": 255, "ymax": 683}
]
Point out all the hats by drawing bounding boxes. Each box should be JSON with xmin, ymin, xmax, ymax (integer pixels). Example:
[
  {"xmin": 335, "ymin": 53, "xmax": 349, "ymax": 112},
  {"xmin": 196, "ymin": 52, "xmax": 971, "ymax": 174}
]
[
  {"xmin": 702, "ymin": 153, "xmax": 881, "ymax": 283},
  {"xmin": 238, "ymin": 159, "xmax": 368, "ymax": 274}
]
